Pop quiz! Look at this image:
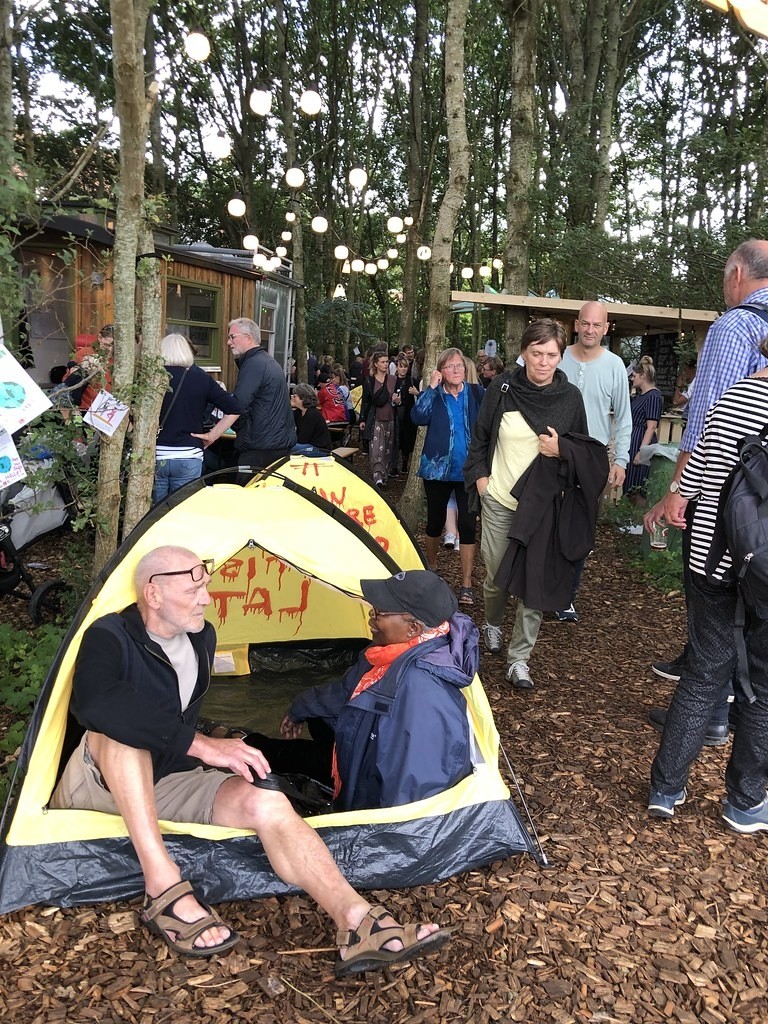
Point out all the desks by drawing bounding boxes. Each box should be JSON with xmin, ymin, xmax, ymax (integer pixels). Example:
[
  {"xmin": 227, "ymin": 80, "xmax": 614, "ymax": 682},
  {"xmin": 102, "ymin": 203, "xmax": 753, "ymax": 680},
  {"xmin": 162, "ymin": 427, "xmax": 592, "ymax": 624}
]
[
  {"xmin": 608, "ymin": 409, "xmax": 685, "ymax": 444},
  {"xmin": 220, "ymin": 433, "xmax": 237, "ymax": 446}
]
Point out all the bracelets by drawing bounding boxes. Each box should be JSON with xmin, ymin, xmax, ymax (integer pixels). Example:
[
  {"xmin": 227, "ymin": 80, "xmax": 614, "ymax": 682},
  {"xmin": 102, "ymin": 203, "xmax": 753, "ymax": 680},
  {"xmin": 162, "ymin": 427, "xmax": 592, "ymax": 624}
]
[{"xmin": 675, "ymin": 385, "xmax": 680, "ymax": 388}]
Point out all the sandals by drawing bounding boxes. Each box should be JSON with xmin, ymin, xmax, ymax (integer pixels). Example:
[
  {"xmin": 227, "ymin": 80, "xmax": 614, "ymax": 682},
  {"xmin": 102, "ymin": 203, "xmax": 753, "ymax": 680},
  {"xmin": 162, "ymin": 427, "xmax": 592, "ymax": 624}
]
[
  {"xmin": 459, "ymin": 586, "xmax": 474, "ymax": 604},
  {"xmin": 335, "ymin": 906, "xmax": 451, "ymax": 976},
  {"xmin": 141, "ymin": 880, "xmax": 239, "ymax": 957}
]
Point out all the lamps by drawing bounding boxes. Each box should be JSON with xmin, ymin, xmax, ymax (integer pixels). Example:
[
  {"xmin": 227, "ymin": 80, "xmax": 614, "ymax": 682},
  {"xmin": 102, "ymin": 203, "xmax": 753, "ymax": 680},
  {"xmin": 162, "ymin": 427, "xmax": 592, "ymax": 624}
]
[
  {"xmin": 493, "ymin": 255, "xmax": 503, "ymax": 269},
  {"xmin": 243, "ymin": 226, "xmax": 292, "ymax": 272},
  {"xmin": 311, "ymin": 210, "xmax": 329, "ymax": 233},
  {"xmin": 403, "ymin": 214, "xmax": 414, "ymax": 225},
  {"xmin": 460, "ymin": 263, "xmax": 474, "ymax": 279},
  {"xmin": 351, "ymin": 255, "xmax": 364, "ymax": 272},
  {"xmin": 365, "ymin": 260, "xmax": 378, "ymax": 275},
  {"xmin": 249, "ymin": 86, "xmax": 272, "ymax": 115},
  {"xmin": 286, "ymin": 160, "xmax": 305, "ymax": 187},
  {"xmin": 416, "ymin": 241, "xmax": 432, "ymax": 260},
  {"xmin": 480, "ymin": 262, "xmax": 491, "ymax": 276},
  {"xmin": 450, "ymin": 260, "xmax": 455, "ymax": 273},
  {"xmin": 349, "ymin": 163, "xmax": 368, "ymax": 186},
  {"xmin": 227, "ymin": 193, "xmax": 246, "ymax": 216},
  {"xmin": 334, "ymin": 240, "xmax": 349, "ymax": 259},
  {"xmin": 378, "ymin": 255, "xmax": 389, "ymax": 269},
  {"xmin": 387, "ymin": 245, "xmax": 398, "ymax": 258},
  {"xmin": 285, "ymin": 209, "xmax": 296, "ymax": 222},
  {"xmin": 397, "ymin": 232, "xmax": 407, "ymax": 243},
  {"xmin": 185, "ymin": 27, "xmax": 211, "ymax": 61},
  {"xmin": 386, "ymin": 210, "xmax": 403, "ymax": 232},
  {"xmin": 300, "ymin": 83, "xmax": 322, "ymax": 115}
]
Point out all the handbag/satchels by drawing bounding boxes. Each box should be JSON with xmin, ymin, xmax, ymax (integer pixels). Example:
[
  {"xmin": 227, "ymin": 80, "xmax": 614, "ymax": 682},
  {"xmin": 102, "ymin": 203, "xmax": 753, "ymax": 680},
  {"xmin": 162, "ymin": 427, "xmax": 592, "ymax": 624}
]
[{"xmin": 374, "ymin": 386, "xmax": 390, "ymax": 407}]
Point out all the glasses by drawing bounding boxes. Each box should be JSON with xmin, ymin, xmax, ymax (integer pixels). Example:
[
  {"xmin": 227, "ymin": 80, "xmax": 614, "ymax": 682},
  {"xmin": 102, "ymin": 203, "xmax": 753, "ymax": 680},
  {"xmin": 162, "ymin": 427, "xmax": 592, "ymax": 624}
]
[
  {"xmin": 373, "ymin": 606, "xmax": 408, "ymax": 621},
  {"xmin": 226, "ymin": 334, "xmax": 247, "ymax": 341},
  {"xmin": 149, "ymin": 559, "xmax": 214, "ymax": 583},
  {"xmin": 442, "ymin": 364, "xmax": 460, "ymax": 370},
  {"xmin": 293, "ymin": 396, "xmax": 297, "ymax": 398}
]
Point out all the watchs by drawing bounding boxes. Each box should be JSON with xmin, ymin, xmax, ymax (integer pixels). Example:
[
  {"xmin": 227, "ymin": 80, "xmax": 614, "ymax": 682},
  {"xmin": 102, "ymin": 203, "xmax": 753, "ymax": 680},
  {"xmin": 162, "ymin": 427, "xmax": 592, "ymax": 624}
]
[{"xmin": 670, "ymin": 481, "xmax": 679, "ymax": 494}]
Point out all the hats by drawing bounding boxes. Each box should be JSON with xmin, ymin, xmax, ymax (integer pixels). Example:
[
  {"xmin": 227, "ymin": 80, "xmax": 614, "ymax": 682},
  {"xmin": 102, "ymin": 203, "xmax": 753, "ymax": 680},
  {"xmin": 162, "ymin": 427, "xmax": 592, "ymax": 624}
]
[
  {"xmin": 360, "ymin": 570, "xmax": 458, "ymax": 628},
  {"xmin": 318, "ymin": 373, "xmax": 331, "ymax": 383}
]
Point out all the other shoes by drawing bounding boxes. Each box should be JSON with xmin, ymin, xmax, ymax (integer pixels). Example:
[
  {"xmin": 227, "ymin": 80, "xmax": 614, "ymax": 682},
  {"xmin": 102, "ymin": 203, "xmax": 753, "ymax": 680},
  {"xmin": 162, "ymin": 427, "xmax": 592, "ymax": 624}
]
[
  {"xmin": 374, "ymin": 472, "xmax": 378, "ymax": 478},
  {"xmin": 444, "ymin": 533, "xmax": 456, "ymax": 547},
  {"xmin": 388, "ymin": 468, "xmax": 399, "ymax": 478},
  {"xmin": 362, "ymin": 450, "xmax": 369, "ymax": 455},
  {"xmin": 195, "ymin": 717, "xmax": 253, "ymax": 741},
  {"xmin": 619, "ymin": 524, "xmax": 643, "ymax": 534},
  {"xmin": 402, "ymin": 465, "xmax": 408, "ymax": 474},
  {"xmin": 375, "ymin": 478, "xmax": 386, "ymax": 486},
  {"xmin": 454, "ymin": 540, "xmax": 460, "ymax": 551}
]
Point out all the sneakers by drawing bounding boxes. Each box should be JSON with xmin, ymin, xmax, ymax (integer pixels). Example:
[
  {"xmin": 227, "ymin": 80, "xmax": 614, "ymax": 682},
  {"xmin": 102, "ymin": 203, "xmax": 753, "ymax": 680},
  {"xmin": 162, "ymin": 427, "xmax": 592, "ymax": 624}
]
[
  {"xmin": 647, "ymin": 787, "xmax": 686, "ymax": 818},
  {"xmin": 556, "ymin": 603, "xmax": 579, "ymax": 622},
  {"xmin": 505, "ymin": 662, "xmax": 534, "ymax": 689},
  {"xmin": 651, "ymin": 655, "xmax": 688, "ymax": 681},
  {"xmin": 721, "ymin": 790, "xmax": 768, "ymax": 833},
  {"xmin": 482, "ymin": 618, "xmax": 503, "ymax": 652},
  {"xmin": 727, "ymin": 679, "xmax": 735, "ymax": 703}
]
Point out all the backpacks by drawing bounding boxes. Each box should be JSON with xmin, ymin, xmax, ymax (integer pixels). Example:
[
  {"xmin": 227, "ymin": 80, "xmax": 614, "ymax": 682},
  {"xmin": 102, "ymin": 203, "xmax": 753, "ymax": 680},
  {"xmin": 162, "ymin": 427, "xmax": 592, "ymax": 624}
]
[{"xmin": 704, "ymin": 424, "xmax": 768, "ymax": 621}]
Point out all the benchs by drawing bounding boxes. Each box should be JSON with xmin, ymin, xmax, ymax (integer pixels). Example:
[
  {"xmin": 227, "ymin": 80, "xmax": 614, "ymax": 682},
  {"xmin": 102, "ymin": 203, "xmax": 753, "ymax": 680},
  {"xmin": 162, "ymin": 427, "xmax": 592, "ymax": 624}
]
[{"xmin": 332, "ymin": 446, "xmax": 359, "ymax": 465}]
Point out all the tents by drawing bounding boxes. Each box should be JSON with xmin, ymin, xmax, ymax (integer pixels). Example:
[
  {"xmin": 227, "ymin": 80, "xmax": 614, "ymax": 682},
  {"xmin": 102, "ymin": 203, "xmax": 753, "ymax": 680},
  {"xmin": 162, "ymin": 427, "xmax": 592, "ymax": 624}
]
[
  {"xmin": 0, "ymin": 456, "xmax": 547, "ymax": 915},
  {"xmin": 350, "ymin": 385, "xmax": 362, "ymax": 414}
]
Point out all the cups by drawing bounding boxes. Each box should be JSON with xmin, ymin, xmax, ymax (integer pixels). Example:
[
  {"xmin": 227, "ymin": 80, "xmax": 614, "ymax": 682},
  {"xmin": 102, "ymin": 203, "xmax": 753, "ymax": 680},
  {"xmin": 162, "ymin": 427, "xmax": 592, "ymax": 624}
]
[{"xmin": 650, "ymin": 520, "xmax": 669, "ymax": 552}]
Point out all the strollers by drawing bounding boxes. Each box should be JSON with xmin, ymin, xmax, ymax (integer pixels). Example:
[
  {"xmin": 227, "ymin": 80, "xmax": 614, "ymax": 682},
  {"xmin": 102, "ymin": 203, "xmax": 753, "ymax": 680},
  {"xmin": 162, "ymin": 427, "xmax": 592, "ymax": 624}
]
[{"xmin": 0, "ymin": 446, "xmax": 80, "ymax": 631}]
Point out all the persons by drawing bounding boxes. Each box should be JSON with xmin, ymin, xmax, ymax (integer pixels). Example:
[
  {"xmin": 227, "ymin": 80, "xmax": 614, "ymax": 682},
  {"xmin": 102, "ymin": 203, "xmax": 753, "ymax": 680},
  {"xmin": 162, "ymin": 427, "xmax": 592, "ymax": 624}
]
[
  {"xmin": 196, "ymin": 570, "xmax": 478, "ymax": 813},
  {"xmin": 153, "ymin": 333, "xmax": 242, "ymax": 507},
  {"xmin": 308, "ymin": 354, "xmax": 356, "ymax": 447},
  {"xmin": 47, "ymin": 545, "xmax": 451, "ymax": 981},
  {"xmin": 647, "ymin": 240, "xmax": 768, "ymax": 745},
  {"xmin": 226, "ymin": 317, "xmax": 298, "ymax": 488},
  {"xmin": 556, "ymin": 302, "xmax": 632, "ymax": 622},
  {"xmin": 293, "ymin": 383, "xmax": 331, "ymax": 448},
  {"xmin": 408, "ymin": 348, "xmax": 503, "ymax": 604},
  {"xmin": 69, "ymin": 324, "xmax": 114, "ymax": 445},
  {"xmin": 622, "ymin": 356, "xmax": 662, "ymax": 507},
  {"xmin": 647, "ymin": 336, "xmax": 768, "ymax": 832},
  {"xmin": 352, "ymin": 341, "xmax": 424, "ymax": 486},
  {"xmin": 463, "ymin": 318, "xmax": 589, "ymax": 689}
]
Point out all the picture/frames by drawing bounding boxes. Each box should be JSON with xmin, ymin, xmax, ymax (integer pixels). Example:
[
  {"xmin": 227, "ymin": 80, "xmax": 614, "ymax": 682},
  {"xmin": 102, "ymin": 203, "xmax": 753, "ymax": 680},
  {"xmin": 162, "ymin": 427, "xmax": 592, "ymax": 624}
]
[{"xmin": 184, "ymin": 293, "xmax": 215, "ymax": 358}]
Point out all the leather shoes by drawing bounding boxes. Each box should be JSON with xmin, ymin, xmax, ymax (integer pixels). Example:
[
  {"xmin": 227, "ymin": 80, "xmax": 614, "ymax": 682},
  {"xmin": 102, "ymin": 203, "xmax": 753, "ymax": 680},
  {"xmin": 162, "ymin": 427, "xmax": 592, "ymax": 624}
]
[{"xmin": 649, "ymin": 709, "xmax": 728, "ymax": 745}]
